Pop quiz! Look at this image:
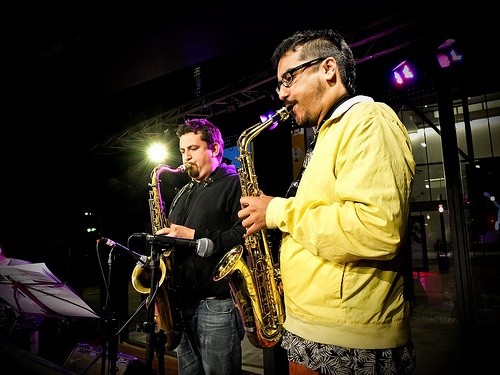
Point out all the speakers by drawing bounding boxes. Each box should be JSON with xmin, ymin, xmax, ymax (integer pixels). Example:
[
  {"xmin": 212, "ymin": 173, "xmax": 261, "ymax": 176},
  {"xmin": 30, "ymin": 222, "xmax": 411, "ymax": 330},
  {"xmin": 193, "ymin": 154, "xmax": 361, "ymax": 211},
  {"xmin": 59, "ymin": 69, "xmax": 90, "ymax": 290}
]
[{"xmin": 62, "ymin": 343, "xmax": 158, "ymax": 375}]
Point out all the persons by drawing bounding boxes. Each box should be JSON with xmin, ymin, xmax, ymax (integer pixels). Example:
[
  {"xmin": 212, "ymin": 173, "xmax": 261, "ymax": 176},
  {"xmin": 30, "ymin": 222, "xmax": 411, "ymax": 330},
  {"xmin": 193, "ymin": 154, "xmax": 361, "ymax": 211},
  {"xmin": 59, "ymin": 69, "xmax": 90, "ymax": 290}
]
[
  {"xmin": 238, "ymin": 29, "xmax": 416, "ymax": 375},
  {"xmin": 155, "ymin": 118, "xmax": 246, "ymax": 375}
]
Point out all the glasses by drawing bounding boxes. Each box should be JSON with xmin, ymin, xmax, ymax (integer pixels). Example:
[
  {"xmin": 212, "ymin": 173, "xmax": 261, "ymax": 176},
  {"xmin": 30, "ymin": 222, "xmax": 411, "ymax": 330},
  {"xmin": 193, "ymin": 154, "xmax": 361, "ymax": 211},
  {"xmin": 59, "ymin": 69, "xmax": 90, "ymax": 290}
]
[{"xmin": 275, "ymin": 55, "xmax": 326, "ymax": 96}]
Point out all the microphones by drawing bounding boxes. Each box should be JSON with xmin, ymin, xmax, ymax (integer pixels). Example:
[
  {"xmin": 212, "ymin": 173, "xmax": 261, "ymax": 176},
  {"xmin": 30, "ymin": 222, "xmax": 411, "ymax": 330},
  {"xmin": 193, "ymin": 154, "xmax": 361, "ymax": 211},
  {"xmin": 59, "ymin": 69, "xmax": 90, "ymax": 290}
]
[
  {"xmin": 102, "ymin": 238, "xmax": 148, "ymax": 267},
  {"xmin": 143, "ymin": 233, "xmax": 214, "ymax": 257}
]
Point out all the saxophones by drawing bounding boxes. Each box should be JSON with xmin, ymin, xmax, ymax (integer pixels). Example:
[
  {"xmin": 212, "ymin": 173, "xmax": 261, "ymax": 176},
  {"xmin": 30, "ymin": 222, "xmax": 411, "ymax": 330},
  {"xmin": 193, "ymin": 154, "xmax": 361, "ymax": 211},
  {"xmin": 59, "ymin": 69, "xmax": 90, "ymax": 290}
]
[
  {"xmin": 213, "ymin": 100, "xmax": 297, "ymax": 348},
  {"xmin": 132, "ymin": 161, "xmax": 195, "ymax": 332}
]
